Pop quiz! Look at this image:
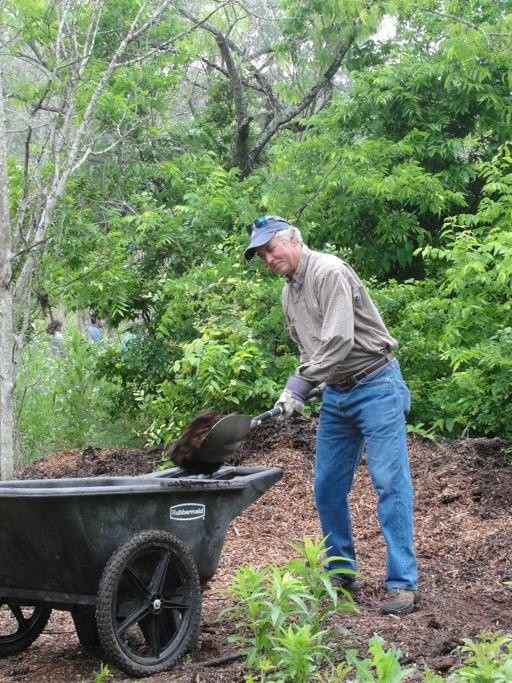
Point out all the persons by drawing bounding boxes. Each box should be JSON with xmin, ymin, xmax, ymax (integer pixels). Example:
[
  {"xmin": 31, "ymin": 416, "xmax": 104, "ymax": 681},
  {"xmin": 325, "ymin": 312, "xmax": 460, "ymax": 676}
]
[
  {"xmin": 244, "ymin": 214, "xmax": 421, "ymax": 616},
  {"xmin": 88, "ymin": 310, "xmax": 105, "ymax": 349},
  {"xmin": 120, "ymin": 323, "xmax": 145, "ymax": 353},
  {"xmin": 44, "ymin": 319, "xmax": 66, "ymax": 361}
]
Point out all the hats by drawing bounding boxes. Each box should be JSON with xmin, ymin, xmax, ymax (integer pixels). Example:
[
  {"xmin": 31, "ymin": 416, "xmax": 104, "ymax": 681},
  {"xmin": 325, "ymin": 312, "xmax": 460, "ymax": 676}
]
[{"xmin": 243, "ymin": 215, "xmax": 291, "ymax": 261}]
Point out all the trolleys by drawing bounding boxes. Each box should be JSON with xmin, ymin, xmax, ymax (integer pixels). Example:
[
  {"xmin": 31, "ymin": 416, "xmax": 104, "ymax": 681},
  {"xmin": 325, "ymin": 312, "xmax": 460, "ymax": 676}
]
[{"xmin": 0, "ymin": 466, "xmax": 284, "ymax": 677}]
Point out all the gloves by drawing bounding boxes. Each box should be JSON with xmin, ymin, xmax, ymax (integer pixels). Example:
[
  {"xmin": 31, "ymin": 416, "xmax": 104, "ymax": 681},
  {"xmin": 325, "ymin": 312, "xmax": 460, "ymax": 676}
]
[
  {"xmin": 307, "ymin": 381, "xmax": 327, "ymax": 404},
  {"xmin": 273, "ymin": 375, "xmax": 315, "ymax": 422}
]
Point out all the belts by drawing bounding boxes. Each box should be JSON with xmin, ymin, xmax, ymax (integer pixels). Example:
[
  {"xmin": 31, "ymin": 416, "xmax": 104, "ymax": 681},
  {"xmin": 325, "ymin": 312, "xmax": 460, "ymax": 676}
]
[{"xmin": 329, "ymin": 353, "xmax": 394, "ymax": 391}]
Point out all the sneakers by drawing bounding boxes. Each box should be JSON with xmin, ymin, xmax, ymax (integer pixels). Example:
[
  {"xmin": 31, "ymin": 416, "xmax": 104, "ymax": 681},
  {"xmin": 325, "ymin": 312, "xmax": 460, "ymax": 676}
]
[
  {"xmin": 317, "ymin": 571, "xmax": 357, "ymax": 598},
  {"xmin": 382, "ymin": 587, "xmax": 419, "ymax": 615}
]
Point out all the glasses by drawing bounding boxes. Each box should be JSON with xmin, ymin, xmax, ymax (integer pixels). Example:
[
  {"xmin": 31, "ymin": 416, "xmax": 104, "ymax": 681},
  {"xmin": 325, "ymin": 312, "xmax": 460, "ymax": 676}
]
[{"xmin": 245, "ymin": 216, "xmax": 267, "ymax": 236}]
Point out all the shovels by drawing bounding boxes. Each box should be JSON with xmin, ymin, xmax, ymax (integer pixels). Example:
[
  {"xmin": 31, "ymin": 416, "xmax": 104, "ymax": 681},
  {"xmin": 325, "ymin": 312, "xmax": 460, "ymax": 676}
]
[{"xmin": 196, "ymin": 384, "xmax": 322, "ymax": 464}]
